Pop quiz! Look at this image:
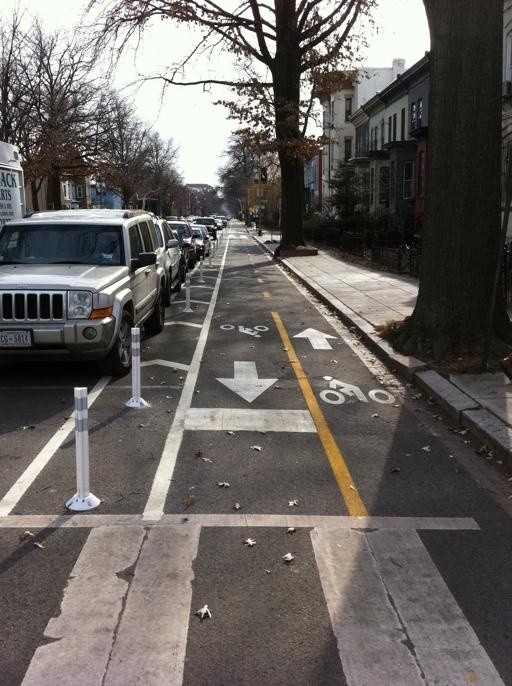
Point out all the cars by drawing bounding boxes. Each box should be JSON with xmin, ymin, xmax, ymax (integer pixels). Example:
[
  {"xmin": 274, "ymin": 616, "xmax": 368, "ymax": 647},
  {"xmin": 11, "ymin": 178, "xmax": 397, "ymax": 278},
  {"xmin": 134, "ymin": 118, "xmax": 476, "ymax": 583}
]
[{"xmin": 190, "ymin": 215, "xmax": 228, "ymax": 261}]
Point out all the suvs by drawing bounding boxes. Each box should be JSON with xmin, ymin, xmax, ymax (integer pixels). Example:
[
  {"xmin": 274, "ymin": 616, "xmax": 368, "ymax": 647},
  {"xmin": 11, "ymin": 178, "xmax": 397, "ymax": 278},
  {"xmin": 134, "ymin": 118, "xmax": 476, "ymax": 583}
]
[{"xmin": 1, "ymin": 209, "xmax": 199, "ymax": 372}]
